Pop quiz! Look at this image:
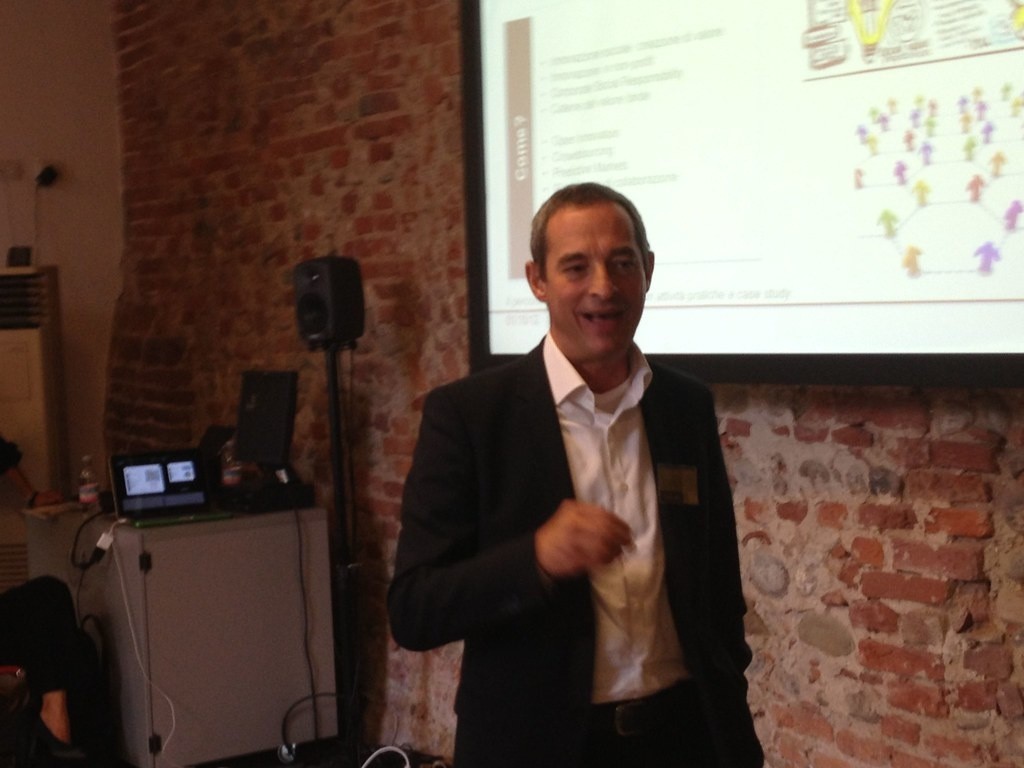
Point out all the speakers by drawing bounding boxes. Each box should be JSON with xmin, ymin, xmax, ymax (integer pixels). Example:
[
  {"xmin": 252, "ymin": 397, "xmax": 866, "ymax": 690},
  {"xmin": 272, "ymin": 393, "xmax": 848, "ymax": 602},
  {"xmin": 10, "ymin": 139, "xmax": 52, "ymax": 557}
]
[{"xmin": 291, "ymin": 257, "xmax": 366, "ymax": 351}]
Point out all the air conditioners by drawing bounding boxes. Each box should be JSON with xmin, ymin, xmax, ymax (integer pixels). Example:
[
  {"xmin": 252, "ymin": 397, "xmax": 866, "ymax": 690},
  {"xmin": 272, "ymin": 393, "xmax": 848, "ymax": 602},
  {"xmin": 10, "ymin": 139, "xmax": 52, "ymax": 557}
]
[{"xmin": 0, "ymin": 265, "xmax": 71, "ymax": 593}]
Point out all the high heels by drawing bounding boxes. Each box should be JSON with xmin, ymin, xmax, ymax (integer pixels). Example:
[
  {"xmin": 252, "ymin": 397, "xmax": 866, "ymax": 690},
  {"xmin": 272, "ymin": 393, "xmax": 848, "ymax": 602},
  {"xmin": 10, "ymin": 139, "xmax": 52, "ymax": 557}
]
[{"xmin": 27, "ymin": 715, "xmax": 89, "ymax": 761}]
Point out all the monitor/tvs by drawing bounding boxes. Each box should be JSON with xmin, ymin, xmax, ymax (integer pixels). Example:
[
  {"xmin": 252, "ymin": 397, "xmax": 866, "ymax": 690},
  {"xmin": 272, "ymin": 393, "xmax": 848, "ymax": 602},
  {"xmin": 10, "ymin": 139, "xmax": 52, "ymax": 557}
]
[
  {"xmin": 107, "ymin": 448, "xmax": 209, "ymax": 522},
  {"xmin": 231, "ymin": 367, "xmax": 298, "ymax": 469}
]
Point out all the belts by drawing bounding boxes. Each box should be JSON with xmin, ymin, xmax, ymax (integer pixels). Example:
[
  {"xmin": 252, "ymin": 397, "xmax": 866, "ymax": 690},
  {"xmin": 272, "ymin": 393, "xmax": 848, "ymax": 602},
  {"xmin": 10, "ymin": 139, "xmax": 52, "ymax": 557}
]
[{"xmin": 589, "ymin": 680, "xmax": 691, "ymax": 745}]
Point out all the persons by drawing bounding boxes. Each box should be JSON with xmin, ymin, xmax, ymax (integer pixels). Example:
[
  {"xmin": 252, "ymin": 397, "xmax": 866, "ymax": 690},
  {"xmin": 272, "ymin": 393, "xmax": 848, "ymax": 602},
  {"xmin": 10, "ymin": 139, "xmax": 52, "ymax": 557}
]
[
  {"xmin": 0, "ymin": 436, "xmax": 108, "ymax": 768},
  {"xmin": 384, "ymin": 180, "xmax": 764, "ymax": 768}
]
